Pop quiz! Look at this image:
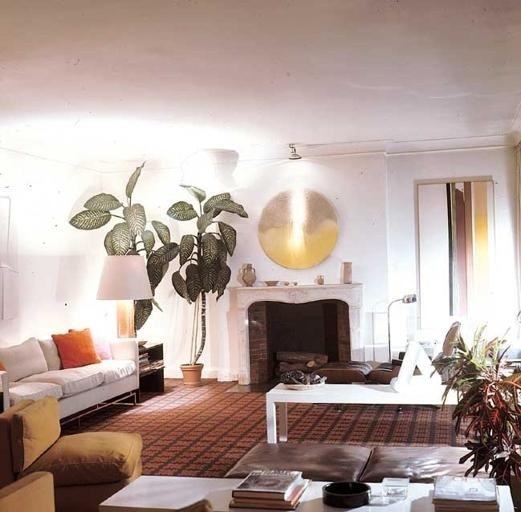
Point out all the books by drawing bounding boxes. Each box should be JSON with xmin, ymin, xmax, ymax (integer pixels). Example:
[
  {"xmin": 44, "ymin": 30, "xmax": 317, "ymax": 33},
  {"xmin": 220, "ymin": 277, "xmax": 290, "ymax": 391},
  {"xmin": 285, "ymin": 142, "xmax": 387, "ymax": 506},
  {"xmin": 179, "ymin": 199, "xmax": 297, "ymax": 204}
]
[
  {"xmin": 431, "ymin": 474, "xmax": 500, "ymax": 512},
  {"xmin": 229, "ymin": 470, "xmax": 312, "ymax": 511}
]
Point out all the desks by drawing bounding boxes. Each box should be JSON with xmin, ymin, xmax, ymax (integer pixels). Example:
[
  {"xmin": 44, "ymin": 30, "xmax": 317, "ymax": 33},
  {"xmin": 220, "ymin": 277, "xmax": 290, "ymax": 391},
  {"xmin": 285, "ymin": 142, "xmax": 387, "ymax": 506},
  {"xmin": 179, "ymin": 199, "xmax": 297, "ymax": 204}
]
[{"xmin": 98, "ymin": 475, "xmax": 515, "ymax": 512}]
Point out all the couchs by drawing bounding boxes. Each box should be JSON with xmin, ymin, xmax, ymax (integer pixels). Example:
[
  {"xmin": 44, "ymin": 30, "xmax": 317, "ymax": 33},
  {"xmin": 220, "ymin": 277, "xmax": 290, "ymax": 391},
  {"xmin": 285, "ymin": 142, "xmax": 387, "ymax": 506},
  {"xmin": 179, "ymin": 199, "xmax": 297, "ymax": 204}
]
[{"xmin": 0, "ymin": 338, "xmax": 141, "ymax": 427}]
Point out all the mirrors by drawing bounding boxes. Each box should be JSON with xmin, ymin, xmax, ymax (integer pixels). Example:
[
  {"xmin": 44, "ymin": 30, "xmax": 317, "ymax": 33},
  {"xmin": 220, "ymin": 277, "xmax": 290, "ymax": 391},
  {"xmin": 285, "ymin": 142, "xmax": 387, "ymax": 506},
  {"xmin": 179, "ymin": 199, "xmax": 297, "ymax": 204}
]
[{"xmin": 413, "ymin": 174, "xmax": 497, "ymax": 336}]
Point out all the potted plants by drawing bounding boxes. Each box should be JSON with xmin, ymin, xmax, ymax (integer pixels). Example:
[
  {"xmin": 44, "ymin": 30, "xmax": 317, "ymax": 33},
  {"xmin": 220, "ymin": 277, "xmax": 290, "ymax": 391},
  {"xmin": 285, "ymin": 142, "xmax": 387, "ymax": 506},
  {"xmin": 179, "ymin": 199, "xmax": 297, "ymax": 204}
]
[{"xmin": 147, "ymin": 185, "xmax": 249, "ymax": 385}]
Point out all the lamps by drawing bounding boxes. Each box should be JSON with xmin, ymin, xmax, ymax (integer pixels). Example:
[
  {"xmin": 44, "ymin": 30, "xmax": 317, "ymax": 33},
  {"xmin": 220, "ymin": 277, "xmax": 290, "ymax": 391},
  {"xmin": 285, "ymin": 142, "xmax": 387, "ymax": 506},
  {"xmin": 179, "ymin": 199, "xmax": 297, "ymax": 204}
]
[
  {"xmin": 95, "ymin": 255, "xmax": 154, "ymax": 339},
  {"xmin": 387, "ymin": 294, "xmax": 416, "ymax": 363},
  {"xmin": 287, "ymin": 143, "xmax": 302, "ymax": 161}
]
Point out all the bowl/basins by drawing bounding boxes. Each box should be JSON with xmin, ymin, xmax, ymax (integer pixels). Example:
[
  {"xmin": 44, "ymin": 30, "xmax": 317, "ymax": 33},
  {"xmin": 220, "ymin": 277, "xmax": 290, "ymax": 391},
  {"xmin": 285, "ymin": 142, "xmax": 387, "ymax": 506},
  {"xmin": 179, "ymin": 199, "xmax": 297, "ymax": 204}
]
[
  {"xmin": 321, "ymin": 481, "xmax": 371, "ymax": 509},
  {"xmin": 264, "ymin": 281, "xmax": 278, "ymax": 286}
]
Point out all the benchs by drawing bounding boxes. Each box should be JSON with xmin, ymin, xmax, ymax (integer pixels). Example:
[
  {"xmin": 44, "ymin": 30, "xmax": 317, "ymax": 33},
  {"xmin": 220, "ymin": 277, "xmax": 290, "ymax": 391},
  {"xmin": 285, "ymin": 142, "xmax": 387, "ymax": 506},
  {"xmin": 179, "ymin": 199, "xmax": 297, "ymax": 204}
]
[{"xmin": 265, "ymin": 383, "xmax": 521, "ymax": 445}]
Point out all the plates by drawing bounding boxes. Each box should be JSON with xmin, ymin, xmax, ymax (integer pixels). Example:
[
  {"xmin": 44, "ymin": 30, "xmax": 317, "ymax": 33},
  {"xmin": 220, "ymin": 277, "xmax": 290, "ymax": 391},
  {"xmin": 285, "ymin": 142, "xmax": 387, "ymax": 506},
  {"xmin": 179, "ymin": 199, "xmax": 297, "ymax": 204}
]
[{"xmin": 283, "ymin": 383, "xmax": 326, "ymax": 390}]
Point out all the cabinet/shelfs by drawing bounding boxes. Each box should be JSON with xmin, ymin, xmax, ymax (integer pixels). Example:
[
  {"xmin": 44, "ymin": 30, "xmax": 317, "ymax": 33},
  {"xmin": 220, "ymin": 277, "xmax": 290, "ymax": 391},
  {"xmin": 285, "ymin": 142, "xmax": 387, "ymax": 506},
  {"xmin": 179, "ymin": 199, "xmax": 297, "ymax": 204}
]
[{"xmin": 137, "ymin": 340, "xmax": 164, "ymax": 403}]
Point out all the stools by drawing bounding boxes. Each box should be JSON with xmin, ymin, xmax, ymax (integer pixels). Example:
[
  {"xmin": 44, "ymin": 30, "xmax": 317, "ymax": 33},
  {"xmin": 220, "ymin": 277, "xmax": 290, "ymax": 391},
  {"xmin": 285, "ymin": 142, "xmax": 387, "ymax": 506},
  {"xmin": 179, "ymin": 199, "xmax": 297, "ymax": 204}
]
[
  {"xmin": 225, "ymin": 442, "xmax": 371, "ymax": 483},
  {"xmin": 358, "ymin": 446, "xmax": 521, "ymax": 487}
]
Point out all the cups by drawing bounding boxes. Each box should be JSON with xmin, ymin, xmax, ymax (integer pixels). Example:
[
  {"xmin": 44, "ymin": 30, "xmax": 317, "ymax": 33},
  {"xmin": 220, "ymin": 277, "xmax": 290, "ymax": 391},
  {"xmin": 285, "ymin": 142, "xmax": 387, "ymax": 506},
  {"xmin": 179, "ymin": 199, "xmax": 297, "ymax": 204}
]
[{"xmin": 381, "ymin": 476, "xmax": 411, "ymax": 501}]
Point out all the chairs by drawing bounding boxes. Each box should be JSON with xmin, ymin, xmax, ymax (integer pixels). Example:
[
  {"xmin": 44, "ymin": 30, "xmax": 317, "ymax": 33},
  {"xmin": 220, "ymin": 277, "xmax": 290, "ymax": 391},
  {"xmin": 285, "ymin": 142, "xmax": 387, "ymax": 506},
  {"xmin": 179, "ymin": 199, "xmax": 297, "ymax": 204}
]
[
  {"xmin": 0, "ymin": 399, "xmax": 143, "ymax": 511},
  {"xmin": 310, "ymin": 321, "xmax": 462, "ymax": 413},
  {"xmin": 0, "ymin": 468, "xmax": 57, "ymax": 511}
]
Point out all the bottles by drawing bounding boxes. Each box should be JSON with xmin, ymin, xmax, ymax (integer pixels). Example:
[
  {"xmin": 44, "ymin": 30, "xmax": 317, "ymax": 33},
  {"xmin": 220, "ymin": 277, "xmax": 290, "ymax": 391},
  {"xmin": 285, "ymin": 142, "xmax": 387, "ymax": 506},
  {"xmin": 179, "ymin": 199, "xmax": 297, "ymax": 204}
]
[
  {"xmin": 342, "ymin": 262, "xmax": 352, "ymax": 283},
  {"xmin": 317, "ymin": 275, "xmax": 324, "ymax": 285}
]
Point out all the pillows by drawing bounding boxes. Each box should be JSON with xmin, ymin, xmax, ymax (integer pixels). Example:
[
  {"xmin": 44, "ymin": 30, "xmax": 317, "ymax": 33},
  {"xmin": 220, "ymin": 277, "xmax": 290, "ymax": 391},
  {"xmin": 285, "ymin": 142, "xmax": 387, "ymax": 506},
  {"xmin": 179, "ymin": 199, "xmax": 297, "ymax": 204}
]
[
  {"xmin": 69, "ymin": 328, "xmax": 112, "ymax": 360},
  {"xmin": 0, "ymin": 336, "xmax": 48, "ymax": 381},
  {"xmin": 51, "ymin": 327, "xmax": 101, "ymax": 369},
  {"xmin": 38, "ymin": 337, "xmax": 62, "ymax": 370},
  {"xmin": 11, "ymin": 394, "xmax": 62, "ymax": 473}
]
